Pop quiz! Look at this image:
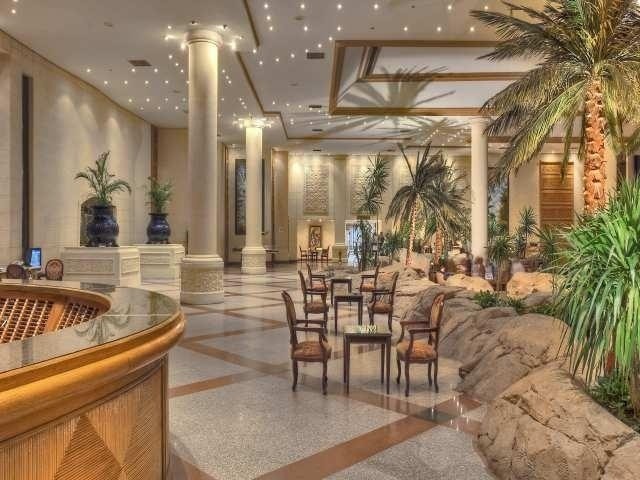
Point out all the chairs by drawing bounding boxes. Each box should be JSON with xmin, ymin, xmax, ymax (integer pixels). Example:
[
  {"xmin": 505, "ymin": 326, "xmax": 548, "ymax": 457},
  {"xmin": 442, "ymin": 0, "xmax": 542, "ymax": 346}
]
[
  {"xmin": 396, "ymin": 295, "xmax": 445, "ymax": 397},
  {"xmin": 307, "ymin": 264, "xmax": 328, "ymax": 291},
  {"xmin": 37, "ymin": 259, "xmax": 64, "ymax": 281},
  {"xmin": 299, "ymin": 246, "xmax": 329, "ymax": 263},
  {"xmin": 7, "ymin": 264, "xmax": 28, "ymax": 279},
  {"xmin": 367, "ymin": 272, "xmax": 399, "ymax": 332},
  {"xmin": 360, "ymin": 262, "xmax": 381, "ymax": 292},
  {"xmin": 281, "ymin": 290, "xmax": 332, "ymax": 395},
  {"xmin": 298, "ymin": 271, "xmax": 329, "ymax": 332}
]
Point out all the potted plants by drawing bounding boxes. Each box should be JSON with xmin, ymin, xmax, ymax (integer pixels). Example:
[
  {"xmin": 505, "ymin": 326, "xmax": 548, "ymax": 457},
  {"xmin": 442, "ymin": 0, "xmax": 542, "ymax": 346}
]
[
  {"xmin": 74, "ymin": 150, "xmax": 132, "ymax": 247},
  {"xmin": 144, "ymin": 176, "xmax": 176, "ymax": 244}
]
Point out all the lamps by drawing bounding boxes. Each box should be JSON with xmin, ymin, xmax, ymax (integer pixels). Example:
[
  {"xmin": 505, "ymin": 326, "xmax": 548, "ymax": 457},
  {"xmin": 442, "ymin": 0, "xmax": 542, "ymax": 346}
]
[{"xmin": 332, "ymin": 243, "xmax": 348, "ymax": 270}]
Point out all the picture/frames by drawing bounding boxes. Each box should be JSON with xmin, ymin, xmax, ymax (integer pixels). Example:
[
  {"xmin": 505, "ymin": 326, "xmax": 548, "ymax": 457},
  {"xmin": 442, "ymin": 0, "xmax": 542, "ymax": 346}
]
[
  {"xmin": 488, "ymin": 167, "xmax": 509, "ymax": 241},
  {"xmin": 235, "ymin": 159, "xmax": 265, "ymax": 236},
  {"xmin": 309, "ymin": 226, "xmax": 321, "ymax": 248}
]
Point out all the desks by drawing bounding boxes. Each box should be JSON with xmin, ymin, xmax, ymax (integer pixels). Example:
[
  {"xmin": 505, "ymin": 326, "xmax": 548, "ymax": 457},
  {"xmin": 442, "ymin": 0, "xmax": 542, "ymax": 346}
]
[
  {"xmin": 0, "ymin": 266, "xmax": 41, "ymax": 279},
  {"xmin": 343, "ymin": 325, "xmax": 392, "ymax": 394},
  {"xmin": 330, "ymin": 277, "xmax": 352, "ymax": 306},
  {"xmin": 334, "ymin": 293, "xmax": 363, "ymax": 336},
  {"xmin": 232, "ymin": 248, "xmax": 280, "ymax": 268}
]
